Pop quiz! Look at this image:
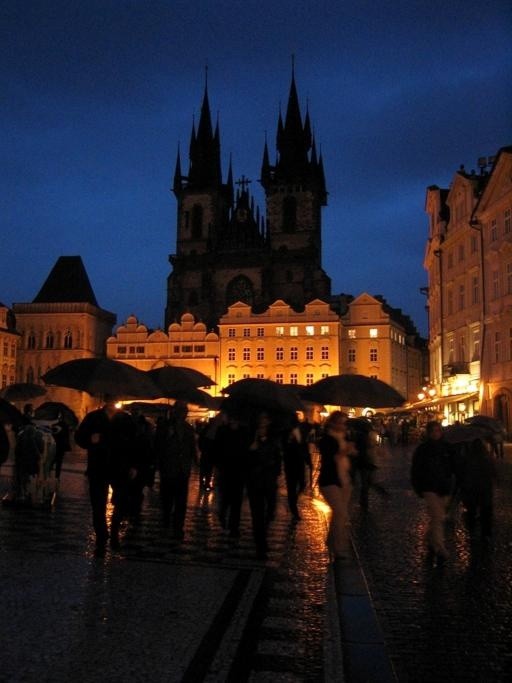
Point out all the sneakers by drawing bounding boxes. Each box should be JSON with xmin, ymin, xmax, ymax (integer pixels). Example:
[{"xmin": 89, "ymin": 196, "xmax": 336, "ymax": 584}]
[{"xmin": 425, "ymin": 541, "xmax": 450, "ymax": 567}]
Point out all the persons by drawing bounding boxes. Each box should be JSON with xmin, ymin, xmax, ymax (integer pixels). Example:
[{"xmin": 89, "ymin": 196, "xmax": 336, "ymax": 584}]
[
  {"xmin": 1, "ymin": 400, "xmax": 389, "ymax": 568},
  {"xmin": 383, "ymin": 415, "xmax": 410, "ymax": 445},
  {"xmin": 487, "ymin": 424, "xmax": 505, "ymax": 459},
  {"xmin": 409, "ymin": 422, "xmax": 499, "ymax": 573}
]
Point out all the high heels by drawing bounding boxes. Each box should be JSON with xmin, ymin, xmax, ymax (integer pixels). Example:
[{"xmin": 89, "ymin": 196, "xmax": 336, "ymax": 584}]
[{"xmin": 326, "ymin": 542, "xmax": 347, "ymax": 562}]
